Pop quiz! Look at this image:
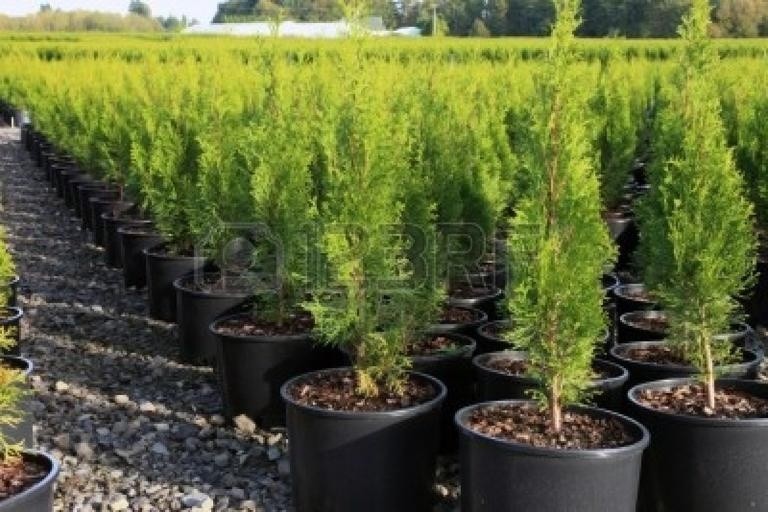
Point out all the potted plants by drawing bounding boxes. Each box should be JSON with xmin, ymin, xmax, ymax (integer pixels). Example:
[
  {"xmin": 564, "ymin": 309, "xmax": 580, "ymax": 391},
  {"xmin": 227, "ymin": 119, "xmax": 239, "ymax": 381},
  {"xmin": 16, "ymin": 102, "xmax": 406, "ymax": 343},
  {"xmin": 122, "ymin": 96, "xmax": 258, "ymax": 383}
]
[{"xmin": 1, "ymin": 0, "xmax": 768, "ymax": 512}]
